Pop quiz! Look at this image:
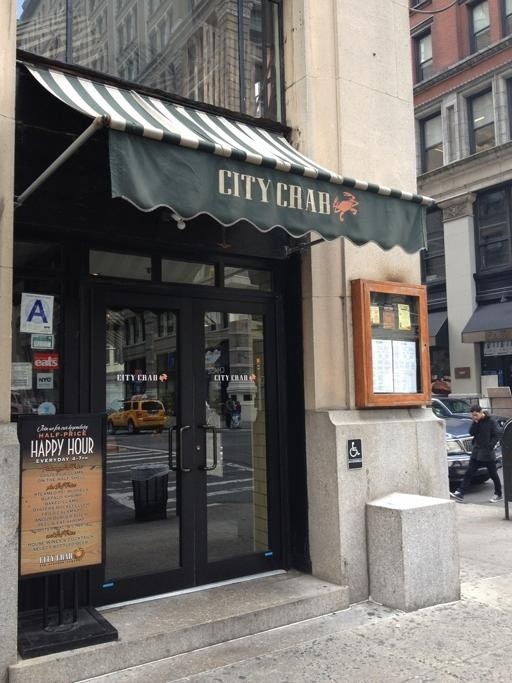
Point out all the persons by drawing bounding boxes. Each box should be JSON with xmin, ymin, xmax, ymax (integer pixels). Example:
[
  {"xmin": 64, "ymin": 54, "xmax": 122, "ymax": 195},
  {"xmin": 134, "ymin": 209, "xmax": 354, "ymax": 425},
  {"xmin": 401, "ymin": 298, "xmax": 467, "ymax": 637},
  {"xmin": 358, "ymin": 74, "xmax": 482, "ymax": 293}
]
[
  {"xmin": 224, "ymin": 395, "xmax": 243, "ymax": 430},
  {"xmin": 448, "ymin": 404, "xmax": 504, "ymax": 504}
]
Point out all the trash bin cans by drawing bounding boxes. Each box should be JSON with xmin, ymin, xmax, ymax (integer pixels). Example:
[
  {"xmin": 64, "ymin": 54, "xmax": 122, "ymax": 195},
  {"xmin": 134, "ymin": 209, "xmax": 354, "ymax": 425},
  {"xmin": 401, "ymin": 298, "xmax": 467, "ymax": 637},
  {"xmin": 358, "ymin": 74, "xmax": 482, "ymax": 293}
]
[{"xmin": 130, "ymin": 465, "xmax": 169, "ymax": 522}]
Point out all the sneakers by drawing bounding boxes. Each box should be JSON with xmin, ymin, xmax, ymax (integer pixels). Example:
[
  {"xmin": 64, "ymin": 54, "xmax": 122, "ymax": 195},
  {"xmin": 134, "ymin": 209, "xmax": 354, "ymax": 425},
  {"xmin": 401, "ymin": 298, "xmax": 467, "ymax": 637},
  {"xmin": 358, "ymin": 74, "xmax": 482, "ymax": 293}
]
[
  {"xmin": 489, "ymin": 494, "xmax": 503, "ymax": 503},
  {"xmin": 449, "ymin": 491, "xmax": 464, "ymax": 500}
]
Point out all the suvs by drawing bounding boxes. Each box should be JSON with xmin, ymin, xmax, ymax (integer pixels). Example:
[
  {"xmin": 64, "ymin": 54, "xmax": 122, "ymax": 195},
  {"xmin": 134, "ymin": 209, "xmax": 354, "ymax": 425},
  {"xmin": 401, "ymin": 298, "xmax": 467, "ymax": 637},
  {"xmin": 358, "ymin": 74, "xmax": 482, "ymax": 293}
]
[{"xmin": 107, "ymin": 392, "xmax": 169, "ymax": 434}]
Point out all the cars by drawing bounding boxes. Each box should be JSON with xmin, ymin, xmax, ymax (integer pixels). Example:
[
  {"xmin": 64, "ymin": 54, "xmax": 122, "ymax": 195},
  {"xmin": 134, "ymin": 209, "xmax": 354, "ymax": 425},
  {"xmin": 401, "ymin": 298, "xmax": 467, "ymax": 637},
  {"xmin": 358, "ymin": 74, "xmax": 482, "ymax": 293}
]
[
  {"xmin": 431, "ymin": 398, "xmax": 504, "ymax": 485},
  {"xmin": 429, "ymin": 394, "xmax": 508, "ymax": 433}
]
